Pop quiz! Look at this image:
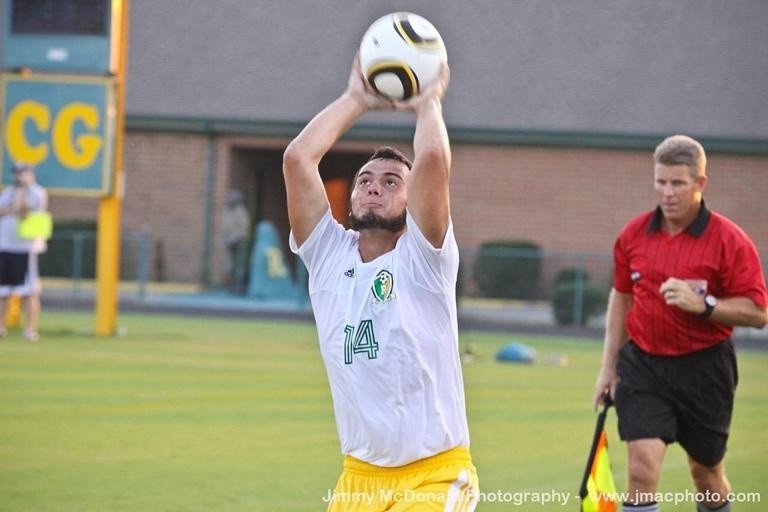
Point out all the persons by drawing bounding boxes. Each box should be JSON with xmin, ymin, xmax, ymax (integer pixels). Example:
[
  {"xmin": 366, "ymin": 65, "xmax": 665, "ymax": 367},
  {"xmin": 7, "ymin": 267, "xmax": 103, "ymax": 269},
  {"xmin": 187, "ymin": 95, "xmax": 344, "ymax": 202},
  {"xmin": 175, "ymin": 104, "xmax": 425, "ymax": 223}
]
[
  {"xmin": 0, "ymin": 161, "xmax": 52, "ymax": 344},
  {"xmin": 590, "ymin": 133, "xmax": 767, "ymax": 512},
  {"xmin": 221, "ymin": 187, "xmax": 252, "ymax": 295},
  {"xmin": 280, "ymin": 45, "xmax": 480, "ymax": 512}
]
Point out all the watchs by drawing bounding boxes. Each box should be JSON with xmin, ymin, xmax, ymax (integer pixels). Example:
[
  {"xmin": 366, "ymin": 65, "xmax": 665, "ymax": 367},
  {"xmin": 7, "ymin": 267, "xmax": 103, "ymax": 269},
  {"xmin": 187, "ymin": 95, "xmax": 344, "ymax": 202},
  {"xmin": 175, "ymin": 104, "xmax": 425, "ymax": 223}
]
[{"xmin": 698, "ymin": 294, "xmax": 716, "ymax": 320}]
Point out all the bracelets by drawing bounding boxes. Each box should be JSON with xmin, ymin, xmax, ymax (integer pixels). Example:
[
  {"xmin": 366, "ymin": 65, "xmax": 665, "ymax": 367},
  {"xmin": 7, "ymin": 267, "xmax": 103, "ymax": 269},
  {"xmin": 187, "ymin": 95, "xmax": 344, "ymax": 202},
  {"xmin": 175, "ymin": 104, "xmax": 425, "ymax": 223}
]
[{"xmin": 14, "ymin": 180, "xmax": 23, "ymax": 188}]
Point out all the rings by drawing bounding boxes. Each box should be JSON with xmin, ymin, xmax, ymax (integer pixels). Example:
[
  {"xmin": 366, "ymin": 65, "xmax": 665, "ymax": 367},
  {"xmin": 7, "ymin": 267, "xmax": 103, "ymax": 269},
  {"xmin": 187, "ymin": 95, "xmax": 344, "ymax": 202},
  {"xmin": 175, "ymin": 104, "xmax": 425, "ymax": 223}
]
[{"xmin": 669, "ymin": 290, "xmax": 673, "ymax": 298}]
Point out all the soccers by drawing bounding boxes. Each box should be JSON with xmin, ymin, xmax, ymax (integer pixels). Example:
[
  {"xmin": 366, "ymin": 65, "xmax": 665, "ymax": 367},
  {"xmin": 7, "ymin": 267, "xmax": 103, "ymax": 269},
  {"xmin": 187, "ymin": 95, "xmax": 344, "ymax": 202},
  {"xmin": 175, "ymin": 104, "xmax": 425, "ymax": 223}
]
[{"xmin": 358, "ymin": 11, "xmax": 448, "ymax": 103}]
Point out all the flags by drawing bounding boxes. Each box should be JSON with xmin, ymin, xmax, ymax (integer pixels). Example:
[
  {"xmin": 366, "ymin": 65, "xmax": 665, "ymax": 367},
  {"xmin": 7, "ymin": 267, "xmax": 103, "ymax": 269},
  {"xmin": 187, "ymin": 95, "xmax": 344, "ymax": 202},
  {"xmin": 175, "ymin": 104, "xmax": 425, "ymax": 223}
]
[{"xmin": 581, "ymin": 430, "xmax": 618, "ymax": 512}]
